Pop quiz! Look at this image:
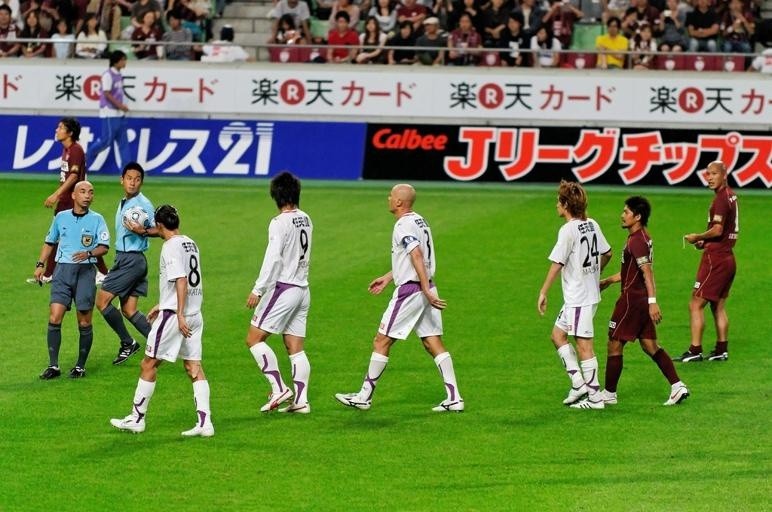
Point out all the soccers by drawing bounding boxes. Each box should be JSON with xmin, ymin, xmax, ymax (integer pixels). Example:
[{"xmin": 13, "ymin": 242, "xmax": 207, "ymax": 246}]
[{"xmin": 122, "ymin": 207, "xmax": 150, "ymax": 231}]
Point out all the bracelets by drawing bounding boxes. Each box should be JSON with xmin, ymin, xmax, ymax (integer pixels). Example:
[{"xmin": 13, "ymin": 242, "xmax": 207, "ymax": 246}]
[
  {"xmin": 86, "ymin": 249, "xmax": 92, "ymax": 259},
  {"xmin": 646, "ymin": 296, "xmax": 658, "ymax": 304},
  {"xmin": 141, "ymin": 228, "xmax": 149, "ymax": 237}
]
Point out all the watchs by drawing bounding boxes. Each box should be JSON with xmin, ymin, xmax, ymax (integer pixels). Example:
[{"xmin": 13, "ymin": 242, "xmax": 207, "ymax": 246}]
[{"xmin": 35, "ymin": 261, "xmax": 44, "ymax": 268}]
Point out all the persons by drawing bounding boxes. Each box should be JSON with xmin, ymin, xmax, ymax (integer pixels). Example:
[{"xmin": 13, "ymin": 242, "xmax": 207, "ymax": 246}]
[
  {"xmin": 537, "ymin": 180, "xmax": 613, "ymax": 409},
  {"xmin": 334, "ymin": 183, "xmax": 467, "ymax": 413},
  {"xmin": 1, "ymin": 0, "xmax": 225, "ymax": 61},
  {"xmin": 32, "ymin": 181, "xmax": 108, "ymax": 381},
  {"xmin": 96, "ymin": 163, "xmax": 165, "ymax": 366},
  {"xmin": 25, "ymin": 118, "xmax": 108, "ymax": 286},
  {"xmin": 265, "ymin": 1, "xmax": 771, "ymax": 72},
  {"xmin": 672, "ymin": 160, "xmax": 739, "ymax": 363},
  {"xmin": 598, "ymin": 196, "xmax": 691, "ymax": 407},
  {"xmin": 110, "ymin": 204, "xmax": 214, "ymax": 438},
  {"xmin": 81, "ymin": 50, "xmax": 133, "ymax": 182},
  {"xmin": 192, "ymin": 24, "xmax": 257, "ymax": 62},
  {"xmin": 244, "ymin": 172, "xmax": 316, "ymax": 414}
]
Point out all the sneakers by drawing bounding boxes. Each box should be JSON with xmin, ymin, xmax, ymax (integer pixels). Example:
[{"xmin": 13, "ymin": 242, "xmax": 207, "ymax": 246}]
[
  {"xmin": 181, "ymin": 425, "xmax": 214, "ymax": 437},
  {"xmin": 68, "ymin": 367, "xmax": 85, "ymax": 378},
  {"xmin": 563, "ymin": 382, "xmax": 618, "ymax": 410},
  {"xmin": 335, "ymin": 393, "xmax": 372, "ymax": 411},
  {"xmin": 39, "ymin": 365, "xmax": 60, "ymax": 380},
  {"xmin": 110, "ymin": 415, "xmax": 145, "ymax": 434},
  {"xmin": 278, "ymin": 401, "xmax": 310, "ymax": 414},
  {"xmin": 260, "ymin": 386, "xmax": 294, "ymax": 412},
  {"xmin": 432, "ymin": 398, "xmax": 465, "ymax": 412},
  {"xmin": 26, "ymin": 275, "xmax": 53, "ymax": 284},
  {"xmin": 95, "ymin": 269, "xmax": 109, "ymax": 286},
  {"xmin": 663, "ymin": 350, "xmax": 729, "ymax": 407},
  {"xmin": 112, "ymin": 339, "xmax": 141, "ymax": 365}
]
